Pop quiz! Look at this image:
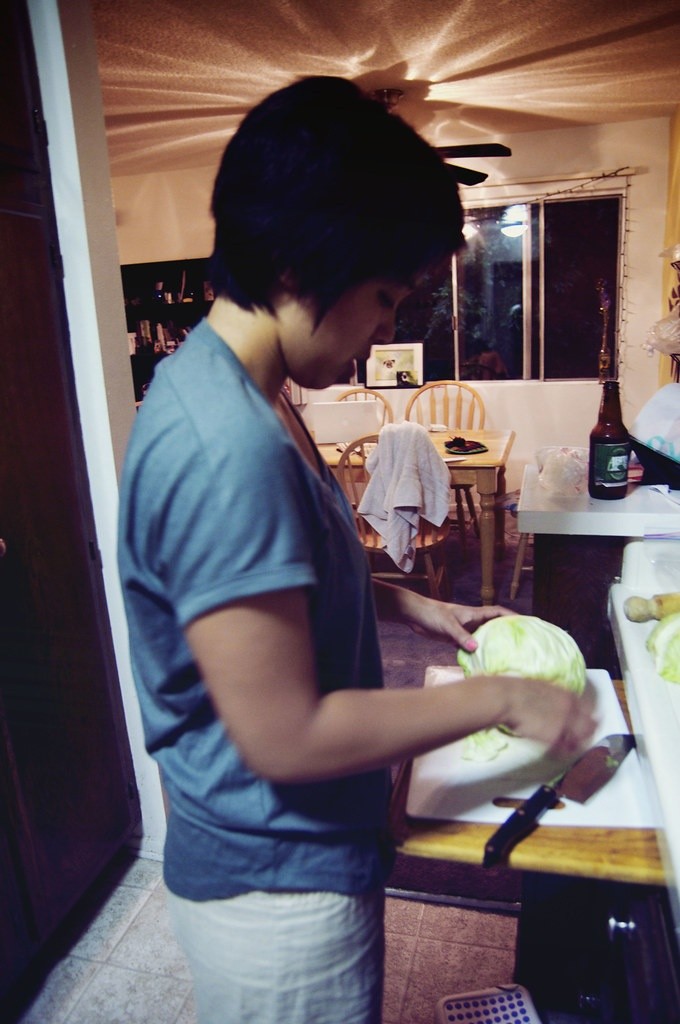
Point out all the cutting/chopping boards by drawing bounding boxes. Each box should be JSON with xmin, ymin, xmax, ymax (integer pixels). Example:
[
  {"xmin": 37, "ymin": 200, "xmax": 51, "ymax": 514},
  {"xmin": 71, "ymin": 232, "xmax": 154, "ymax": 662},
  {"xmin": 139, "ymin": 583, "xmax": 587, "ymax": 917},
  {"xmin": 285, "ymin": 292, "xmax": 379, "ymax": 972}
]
[{"xmin": 406, "ymin": 666, "xmax": 655, "ymax": 827}]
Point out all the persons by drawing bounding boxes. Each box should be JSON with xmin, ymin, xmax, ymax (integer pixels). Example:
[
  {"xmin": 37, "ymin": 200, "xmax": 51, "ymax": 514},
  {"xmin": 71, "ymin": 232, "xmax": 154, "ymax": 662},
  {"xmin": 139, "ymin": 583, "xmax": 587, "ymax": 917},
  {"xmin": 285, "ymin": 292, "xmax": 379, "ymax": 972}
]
[{"xmin": 118, "ymin": 76, "xmax": 596, "ymax": 1024}]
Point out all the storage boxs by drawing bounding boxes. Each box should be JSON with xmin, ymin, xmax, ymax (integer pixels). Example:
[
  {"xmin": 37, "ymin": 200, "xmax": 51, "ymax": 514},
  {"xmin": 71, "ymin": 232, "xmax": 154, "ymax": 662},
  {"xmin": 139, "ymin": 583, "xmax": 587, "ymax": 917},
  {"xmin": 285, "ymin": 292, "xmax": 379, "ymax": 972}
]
[
  {"xmin": 313, "ymin": 400, "xmax": 387, "ymax": 443},
  {"xmin": 203, "ymin": 281, "xmax": 215, "ymax": 300},
  {"xmin": 126, "ymin": 332, "xmax": 137, "ymax": 356}
]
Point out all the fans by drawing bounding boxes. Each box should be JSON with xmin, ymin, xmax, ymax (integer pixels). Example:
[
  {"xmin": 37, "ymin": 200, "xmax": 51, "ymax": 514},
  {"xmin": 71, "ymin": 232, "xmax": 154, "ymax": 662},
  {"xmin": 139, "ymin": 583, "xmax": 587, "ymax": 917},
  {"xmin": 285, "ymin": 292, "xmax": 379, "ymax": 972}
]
[{"xmin": 371, "ymin": 89, "xmax": 512, "ymax": 188}]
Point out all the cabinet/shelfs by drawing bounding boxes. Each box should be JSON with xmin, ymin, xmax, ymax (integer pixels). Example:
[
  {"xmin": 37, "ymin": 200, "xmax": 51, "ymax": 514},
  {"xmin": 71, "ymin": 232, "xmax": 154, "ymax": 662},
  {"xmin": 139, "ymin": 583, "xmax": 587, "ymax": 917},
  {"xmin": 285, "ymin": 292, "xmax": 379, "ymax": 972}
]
[{"xmin": 119, "ymin": 257, "xmax": 218, "ymax": 399}]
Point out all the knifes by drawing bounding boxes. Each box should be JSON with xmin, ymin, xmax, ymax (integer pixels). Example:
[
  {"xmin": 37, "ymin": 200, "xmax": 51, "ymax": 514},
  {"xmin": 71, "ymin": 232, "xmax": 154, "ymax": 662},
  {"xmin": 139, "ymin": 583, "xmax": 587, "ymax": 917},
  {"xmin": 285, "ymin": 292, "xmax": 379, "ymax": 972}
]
[{"xmin": 484, "ymin": 733, "xmax": 635, "ymax": 863}]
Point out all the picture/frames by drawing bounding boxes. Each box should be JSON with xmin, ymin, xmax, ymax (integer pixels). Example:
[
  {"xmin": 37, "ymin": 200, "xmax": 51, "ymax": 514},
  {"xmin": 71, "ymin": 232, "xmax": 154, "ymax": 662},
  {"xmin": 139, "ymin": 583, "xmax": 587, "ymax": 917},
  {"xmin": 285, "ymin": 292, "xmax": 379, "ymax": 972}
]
[{"xmin": 364, "ymin": 340, "xmax": 425, "ymax": 389}]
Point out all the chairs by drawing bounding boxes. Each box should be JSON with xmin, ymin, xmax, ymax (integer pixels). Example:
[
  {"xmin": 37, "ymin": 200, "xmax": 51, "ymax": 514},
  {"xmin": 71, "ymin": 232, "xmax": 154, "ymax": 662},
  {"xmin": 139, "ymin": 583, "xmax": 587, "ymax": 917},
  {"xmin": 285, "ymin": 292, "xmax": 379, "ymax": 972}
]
[
  {"xmin": 405, "ymin": 380, "xmax": 485, "ymax": 430},
  {"xmin": 339, "ymin": 389, "xmax": 394, "ymax": 426},
  {"xmin": 337, "ymin": 433, "xmax": 452, "ymax": 604}
]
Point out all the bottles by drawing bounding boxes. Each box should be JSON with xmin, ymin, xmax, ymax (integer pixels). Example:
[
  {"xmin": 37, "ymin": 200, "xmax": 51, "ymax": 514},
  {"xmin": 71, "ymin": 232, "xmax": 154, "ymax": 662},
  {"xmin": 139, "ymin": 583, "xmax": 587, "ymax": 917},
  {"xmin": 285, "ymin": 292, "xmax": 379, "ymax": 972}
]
[{"xmin": 589, "ymin": 382, "xmax": 631, "ymax": 500}]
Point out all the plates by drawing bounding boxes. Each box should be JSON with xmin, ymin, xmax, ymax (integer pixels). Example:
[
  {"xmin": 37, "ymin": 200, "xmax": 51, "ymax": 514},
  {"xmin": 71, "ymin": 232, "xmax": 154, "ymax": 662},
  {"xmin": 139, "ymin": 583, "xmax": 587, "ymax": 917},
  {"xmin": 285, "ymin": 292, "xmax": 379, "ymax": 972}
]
[{"xmin": 447, "ymin": 446, "xmax": 488, "ymax": 454}]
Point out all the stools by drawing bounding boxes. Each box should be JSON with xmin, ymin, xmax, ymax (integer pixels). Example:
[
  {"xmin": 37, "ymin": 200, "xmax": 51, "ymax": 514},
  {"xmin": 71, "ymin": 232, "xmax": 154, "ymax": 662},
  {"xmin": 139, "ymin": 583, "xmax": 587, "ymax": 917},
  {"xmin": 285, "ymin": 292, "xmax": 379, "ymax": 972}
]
[{"xmin": 498, "ymin": 489, "xmax": 533, "ymax": 601}]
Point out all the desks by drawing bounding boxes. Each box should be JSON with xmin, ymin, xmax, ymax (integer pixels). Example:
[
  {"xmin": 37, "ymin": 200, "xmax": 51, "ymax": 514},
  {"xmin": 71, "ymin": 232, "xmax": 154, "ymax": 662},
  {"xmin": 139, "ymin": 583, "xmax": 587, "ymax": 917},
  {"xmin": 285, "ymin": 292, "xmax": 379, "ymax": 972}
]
[
  {"xmin": 388, "ymin": 678, "xmax": 665, "ymax": 887},
  {"xmin": 307, "ymin": 428, "xmax": 516, "ymax": 606},
  {"xmin": 518, "ymin": 463, "xmax": 680, "ymax": 680}
]
